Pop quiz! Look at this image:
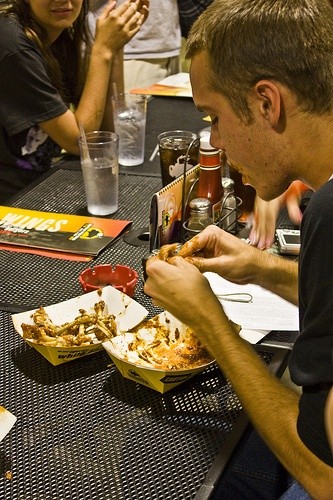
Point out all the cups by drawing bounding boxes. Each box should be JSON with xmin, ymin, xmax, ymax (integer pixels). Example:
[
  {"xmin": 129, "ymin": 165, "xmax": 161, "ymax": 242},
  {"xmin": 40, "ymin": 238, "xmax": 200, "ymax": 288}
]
[
  {"xmin": 111, "ymin": 93, "xmax": 147, "ymax": 166},
  {"xmin": 78, "ymin": 131, "xmax": 119, "ymax": 215},
  {"xmin": 158, "ymin": 130, "xmax": 199, "ymax": 188}
]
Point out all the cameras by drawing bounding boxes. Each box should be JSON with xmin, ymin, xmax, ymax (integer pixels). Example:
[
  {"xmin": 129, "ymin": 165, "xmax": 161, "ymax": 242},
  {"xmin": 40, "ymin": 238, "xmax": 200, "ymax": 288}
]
[{"xmin": 275, "ymin": 229, "xmax": 301, "ymax": 255}]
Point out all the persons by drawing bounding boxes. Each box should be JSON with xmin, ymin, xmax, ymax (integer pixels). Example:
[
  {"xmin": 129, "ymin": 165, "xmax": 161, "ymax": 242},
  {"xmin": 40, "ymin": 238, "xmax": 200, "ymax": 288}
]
[
  {"xmin": 143, "ymin": 0, "xmax": 332, "ymax": 500},
  {"xmin": 0, "ymin": 0, "xmax": 150, "ymax": 206},
  {"xmin": 81, "ymin": 1, "xmax": 181, "ymax": 111},
  {"xmin": 248, "ymin": 180, "xmax": 311, "ymax": 250}
]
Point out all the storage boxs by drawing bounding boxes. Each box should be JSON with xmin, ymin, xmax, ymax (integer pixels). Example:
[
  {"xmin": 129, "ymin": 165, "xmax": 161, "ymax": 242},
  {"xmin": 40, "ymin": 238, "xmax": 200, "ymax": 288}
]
[
  {"xmin": 101, "ymin": 309, "xmax": 242, "ymax": 395},
  {"xmin": 11, "ymin": 285, "xmax": 149, "ymax": 366}
]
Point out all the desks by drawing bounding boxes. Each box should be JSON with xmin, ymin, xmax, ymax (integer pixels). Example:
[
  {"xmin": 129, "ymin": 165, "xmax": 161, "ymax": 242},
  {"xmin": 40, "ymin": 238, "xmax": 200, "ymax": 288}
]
[{"xmin": 0, "ymin": 93, "xmax": 312, "ymax": 500}]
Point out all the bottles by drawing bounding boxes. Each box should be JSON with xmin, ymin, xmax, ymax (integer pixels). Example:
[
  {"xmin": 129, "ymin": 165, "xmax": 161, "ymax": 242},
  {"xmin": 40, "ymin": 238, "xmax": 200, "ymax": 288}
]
[
  {"xmin": 197, "ymin": 137, "xmax": 221, "ymax": 227},
  {"xmin": 187, "ymin": 197, "xmax": 213, "ymax": 238},
  {"xmin": 222, "ymin": 178, "xmax": 236, "ymax": 231}
]
